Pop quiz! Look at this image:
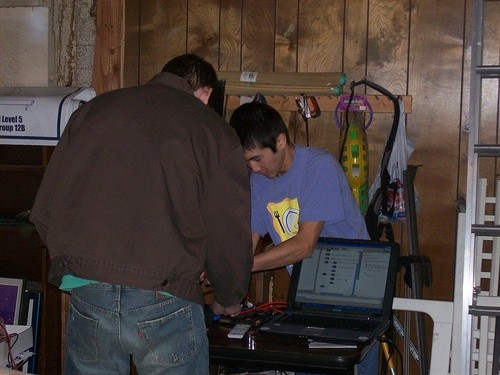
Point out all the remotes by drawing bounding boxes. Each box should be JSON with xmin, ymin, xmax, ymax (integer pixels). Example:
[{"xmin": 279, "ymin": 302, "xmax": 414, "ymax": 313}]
[{"xmin": 228, "ymin": 324, "xmax": 250, "ymax": 339}]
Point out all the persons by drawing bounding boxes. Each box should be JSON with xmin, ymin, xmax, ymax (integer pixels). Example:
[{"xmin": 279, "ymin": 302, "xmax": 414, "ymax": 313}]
[
  {"xmin": 29, "ymin": 53, "xmax": 252, "ymax": 375},
  {"xmin": 200, "ymin": 101, "xmax": 381, "ymax": 375}
]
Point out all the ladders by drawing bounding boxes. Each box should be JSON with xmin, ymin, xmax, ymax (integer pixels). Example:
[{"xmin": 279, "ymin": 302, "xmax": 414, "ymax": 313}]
[{"xmin": 450, "ymin": 0, "xmax": 500, "ymax": 375}]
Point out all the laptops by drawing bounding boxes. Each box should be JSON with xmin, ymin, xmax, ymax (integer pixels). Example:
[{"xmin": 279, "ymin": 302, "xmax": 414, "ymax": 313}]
[{"xmin": 259, "ymin": 237, "xmax": 400, "ymax": 344}]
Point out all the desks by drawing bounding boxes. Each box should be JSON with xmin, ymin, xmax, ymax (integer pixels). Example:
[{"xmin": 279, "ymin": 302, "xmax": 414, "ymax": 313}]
[{"xmin": 211, "ymin": 308, "xmax": 395, "ymax": 375}]
